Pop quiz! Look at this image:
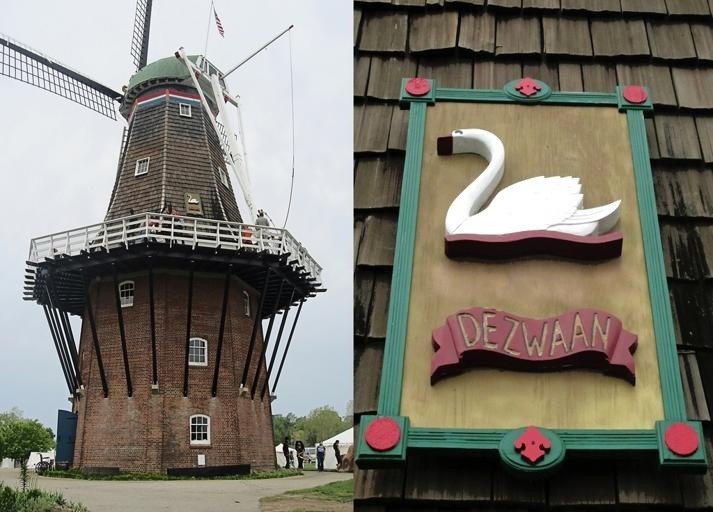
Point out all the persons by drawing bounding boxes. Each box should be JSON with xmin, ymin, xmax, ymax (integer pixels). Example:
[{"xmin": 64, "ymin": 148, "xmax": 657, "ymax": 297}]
[
  {"xmin": 127, "ymin": 209, "xmax": 139, "ymax": 236},
  {"xmin": 161, "ymin": 204, "xmax": 177, "ymax": 237},
  {"xmin": 254, "ymin": 209, "xmax": 269, "ymax": 239},
  {"xmin": 332, "ymin": 440, "xmax": 341, "ymax": 470},
  {"xmin": 289, "ymin": 450, "xmax": 294, "ymax": 468},
  {"xmin": 242, "ymin": 223, "xmax": 254, "ymax": 246},
  {"xmin": 282, "ymin": 436, "xmax": 291, "ymax": 469},
  {"xmin": 249, "ymin": 238, "xmax": 251, "ymax": 238},
  {"xmin": 294, "ymin": 440, "xmax": 306, "ymax": 468},
  {"xmin": 315, "ymin": 440, "xmax": 325, "ymax": 472},
  {"xmin": 34, "ymin": 333, "xmax": 42, "ymax": 336}
]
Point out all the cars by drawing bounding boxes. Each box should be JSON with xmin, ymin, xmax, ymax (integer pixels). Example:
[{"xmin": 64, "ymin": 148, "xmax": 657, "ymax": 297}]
[{"xmin": 303, "ymin": 447, "xmax": 315, "ymax": 464}]
